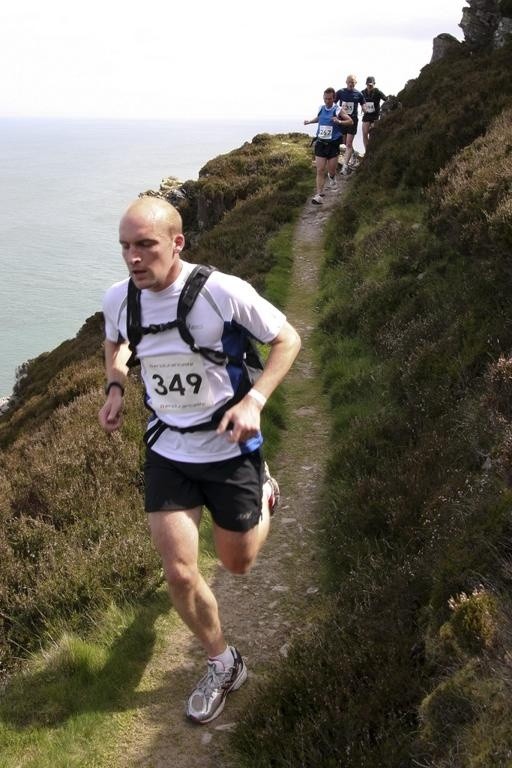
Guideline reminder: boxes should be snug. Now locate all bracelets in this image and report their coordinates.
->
[248,388,267,406]
[104,381,125,397]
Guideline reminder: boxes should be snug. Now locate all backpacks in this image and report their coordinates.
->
[126,263,265,395]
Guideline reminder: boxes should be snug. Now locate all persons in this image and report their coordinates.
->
[304,74,387,204]
[96,193,302,724]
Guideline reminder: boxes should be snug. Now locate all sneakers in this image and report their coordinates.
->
[261,460,280,518]
[328,172,337,187]
[339,150,360,176]
[311,193,326,205]
[185,645,249,726]
